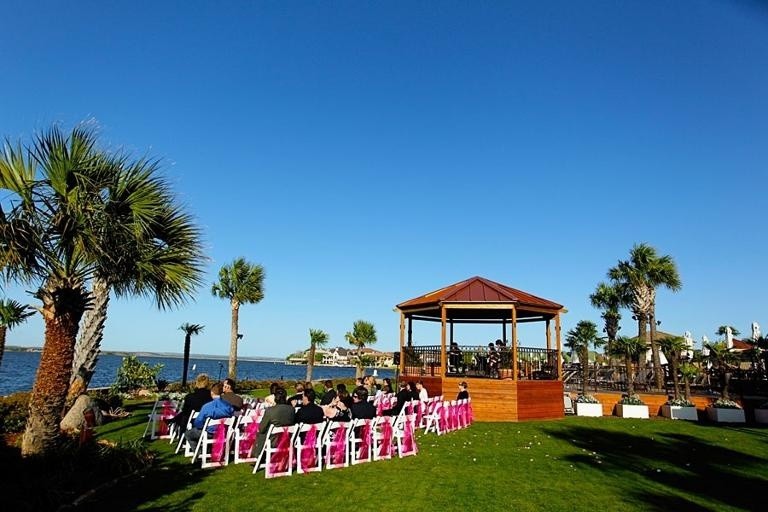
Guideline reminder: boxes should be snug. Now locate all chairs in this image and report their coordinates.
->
[313,419,355,469]
[348,418,376,465]
[226,415,263,464]
[288,420,327,474]
[252,423,299,477]
[364,393,472,434]
[364,415,397,461]
[189,416,236,469]
[447,353,458,373]
[142,393,302,460]
[387,414,419,458]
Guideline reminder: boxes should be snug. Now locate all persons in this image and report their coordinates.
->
[164,373,429,472]
[447,339,508,400]
